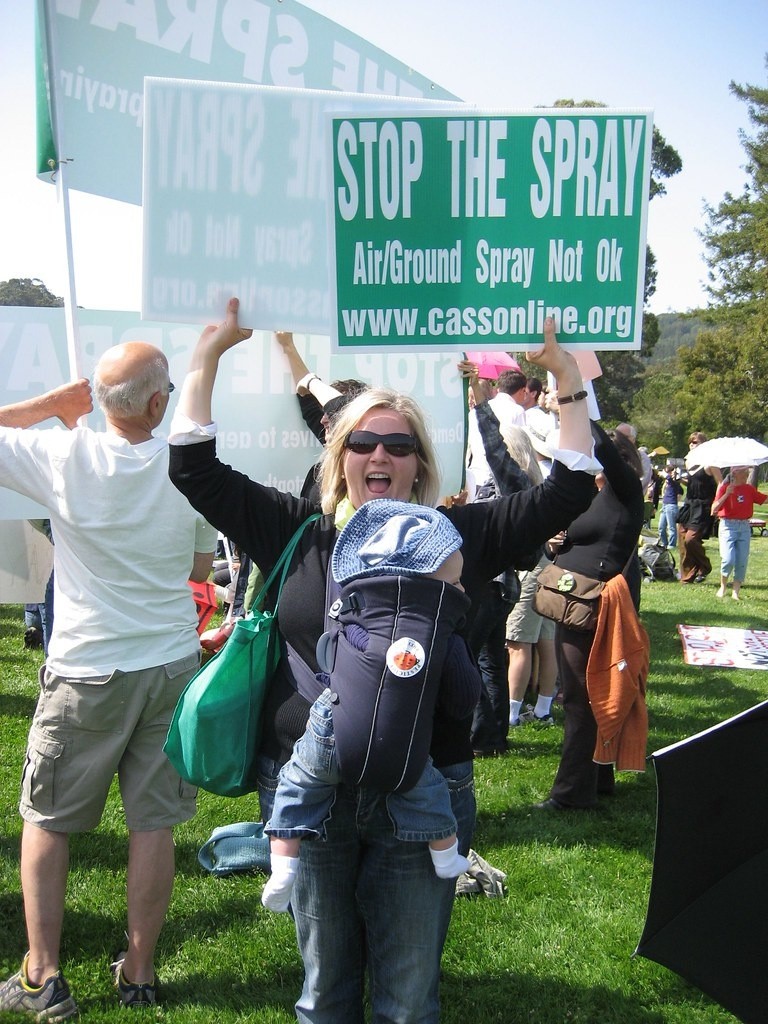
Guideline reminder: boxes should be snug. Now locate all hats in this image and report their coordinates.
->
[541,386,549,395]
[331,499,464,587]
[730,466,754,472]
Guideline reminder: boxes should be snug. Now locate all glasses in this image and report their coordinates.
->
[740,471,751,473]
[626,435,634,438]
[167,382,175,393]
[343,431,419,457]
[689,441,702,444]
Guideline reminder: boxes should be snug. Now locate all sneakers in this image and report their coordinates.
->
[521,712,555,727]
[115,951,157,1010]
[1,950,78,1023]
[509,717,521,727]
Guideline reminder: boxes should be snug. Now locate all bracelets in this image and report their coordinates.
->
[307,375,321,390]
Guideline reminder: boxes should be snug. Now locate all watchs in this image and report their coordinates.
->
[557,391,587,405]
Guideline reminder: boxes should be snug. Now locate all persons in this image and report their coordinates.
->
[0,341,218,1024]
[169,297,604,1024]
[259,497,472,911]
[25,520,55,654]
[710,466,768,602]
[456,370,644,814]
[275,328,372,504]
[605,423,724,583]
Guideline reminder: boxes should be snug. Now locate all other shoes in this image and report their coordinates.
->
[693,576,706,583]
[666,545,675,550]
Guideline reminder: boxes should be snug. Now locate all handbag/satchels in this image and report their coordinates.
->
[161,508,325,797]
[643,543,676,580]
[531,563,608,631]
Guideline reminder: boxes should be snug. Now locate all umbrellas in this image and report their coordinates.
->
[630,701,768,1024]
[684,436,768,508]
[465,351,520,368]
[465,351,521,380]
